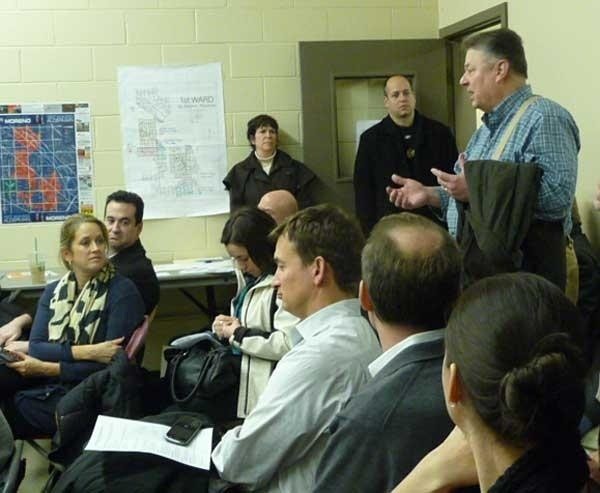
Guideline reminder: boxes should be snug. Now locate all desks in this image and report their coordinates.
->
[0,257,237,322]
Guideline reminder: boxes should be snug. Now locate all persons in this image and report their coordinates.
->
[42,187,600,493]
[384,29,581,297]
[222,113,318,212]
[352,73,459,239]
[2,187,159,492]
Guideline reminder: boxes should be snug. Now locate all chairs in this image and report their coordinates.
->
[2,303,159,493]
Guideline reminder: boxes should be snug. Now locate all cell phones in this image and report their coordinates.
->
[164,414,203,445]
[0,347,24,363]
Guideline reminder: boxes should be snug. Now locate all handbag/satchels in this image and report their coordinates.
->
[565,240,579,306]
[164,339,241,411]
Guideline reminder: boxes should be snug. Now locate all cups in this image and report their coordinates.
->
[26,249,48,285]
